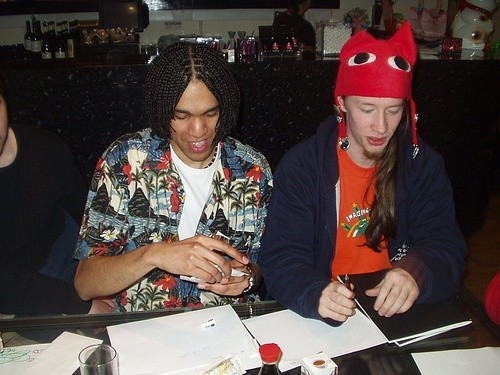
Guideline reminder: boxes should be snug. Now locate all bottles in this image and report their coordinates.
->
[26,20,80,61]
[258,344,283,375]
[261,25,300,56]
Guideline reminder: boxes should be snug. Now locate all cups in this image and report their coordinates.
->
[79,343,119,375]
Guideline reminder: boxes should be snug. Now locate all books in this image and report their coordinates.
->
[336,267,473,348]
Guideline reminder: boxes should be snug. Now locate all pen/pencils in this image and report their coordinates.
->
[345,274,350,289]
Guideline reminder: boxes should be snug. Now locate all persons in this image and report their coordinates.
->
[72,41,273,315]
[272,0,316,54]
[257,21,469,327]
[0,94,92,315]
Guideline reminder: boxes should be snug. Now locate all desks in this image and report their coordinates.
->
[0,285,500,375]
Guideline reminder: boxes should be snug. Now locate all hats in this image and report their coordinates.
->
[334,22,419,159]
